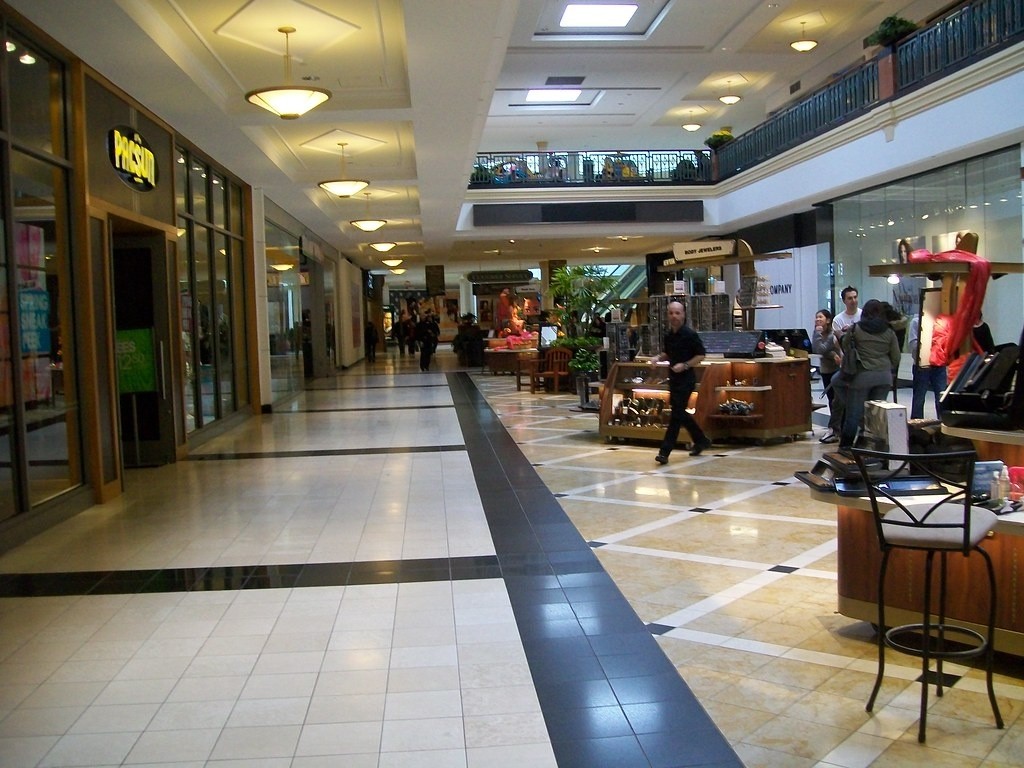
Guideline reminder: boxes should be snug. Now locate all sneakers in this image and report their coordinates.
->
[819,427,839,443]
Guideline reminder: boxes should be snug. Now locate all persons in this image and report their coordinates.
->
[812,286,994,450]
[590,313,607,339]
[364,321,379,363]
[887,239,923,318]
[391,309,441,372]
[649,301,712,464]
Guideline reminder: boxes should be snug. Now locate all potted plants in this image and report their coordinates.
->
[703,130,735,149]
[868,14,918,45]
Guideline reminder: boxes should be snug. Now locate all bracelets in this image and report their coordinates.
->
[657,354,662,361]
[684,362,688,370]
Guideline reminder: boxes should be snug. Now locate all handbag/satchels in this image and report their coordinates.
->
[840,323,858,383]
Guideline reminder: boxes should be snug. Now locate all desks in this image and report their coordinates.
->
[482,338,540,376]
[515,358,549,394]
[809,422,1024,668]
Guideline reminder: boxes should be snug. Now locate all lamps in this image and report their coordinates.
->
[381,252,403,267]
[717,80,741,105]
[790,22,818,52]
[269,242,294,271]
[244,27,333,120]
[317,143,370,198]
[350,193,387,232]
[368,228,397,252]
[681,111,701,132]
[388,267,408,275]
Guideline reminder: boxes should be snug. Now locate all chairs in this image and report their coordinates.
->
[850,447,1005,743]
[530,347,573,395]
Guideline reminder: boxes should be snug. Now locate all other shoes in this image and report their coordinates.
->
[689,437,711,456]
[655,453,669,464]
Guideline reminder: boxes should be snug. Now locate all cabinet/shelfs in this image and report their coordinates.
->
[599,362,772,444]
